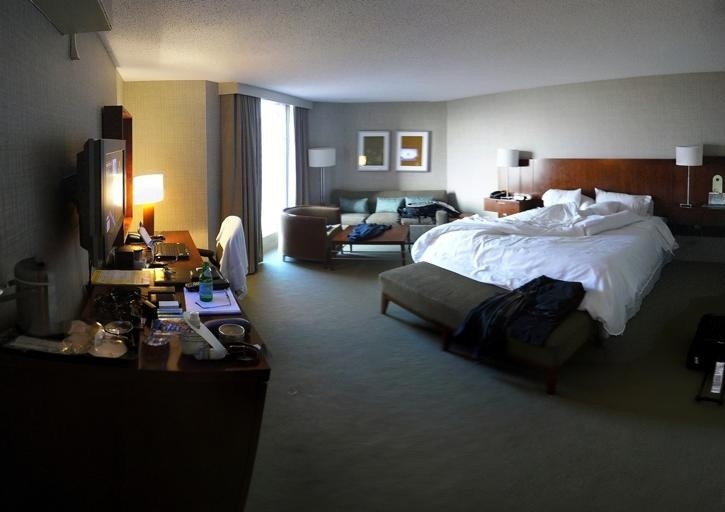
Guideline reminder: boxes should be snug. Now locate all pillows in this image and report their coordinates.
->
[405,196,434,208]
[339,196,369,213]
[595,187,652,219]
[543,188,582,211]
[375,196,404,214]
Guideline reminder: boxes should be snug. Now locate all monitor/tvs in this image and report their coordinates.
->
[75,138,129,263]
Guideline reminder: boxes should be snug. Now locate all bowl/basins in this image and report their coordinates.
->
[218,324,245,340]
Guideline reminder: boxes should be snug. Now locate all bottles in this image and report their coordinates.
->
[200,263,213,302]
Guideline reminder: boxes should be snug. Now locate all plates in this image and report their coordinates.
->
[105,320,133,335]
[86,340,129,358]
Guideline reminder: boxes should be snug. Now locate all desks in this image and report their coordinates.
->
[0,230,270,512]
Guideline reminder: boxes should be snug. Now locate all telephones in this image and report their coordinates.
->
[490,190,510,198]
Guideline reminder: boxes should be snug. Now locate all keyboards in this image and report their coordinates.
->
[152,240,192,260]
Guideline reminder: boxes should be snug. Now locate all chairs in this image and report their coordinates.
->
[195,216,242,299]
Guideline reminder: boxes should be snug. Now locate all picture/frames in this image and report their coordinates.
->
[394,130,430,173]
[356,129,390,173]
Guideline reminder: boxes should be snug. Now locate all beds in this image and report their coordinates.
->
[380,188,676,392]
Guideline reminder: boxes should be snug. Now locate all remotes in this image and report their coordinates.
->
[184,279,230,290]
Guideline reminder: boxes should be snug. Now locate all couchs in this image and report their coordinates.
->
[326,189,450,244]
[279,204,344,269]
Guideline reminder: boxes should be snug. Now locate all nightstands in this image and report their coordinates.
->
[484,195,541,219]
[674,205,724,283]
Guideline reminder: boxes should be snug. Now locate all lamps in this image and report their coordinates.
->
[133,173,164,237]
[308,146,336,205]
[675,144,704,210]
[497,148,520,195]
